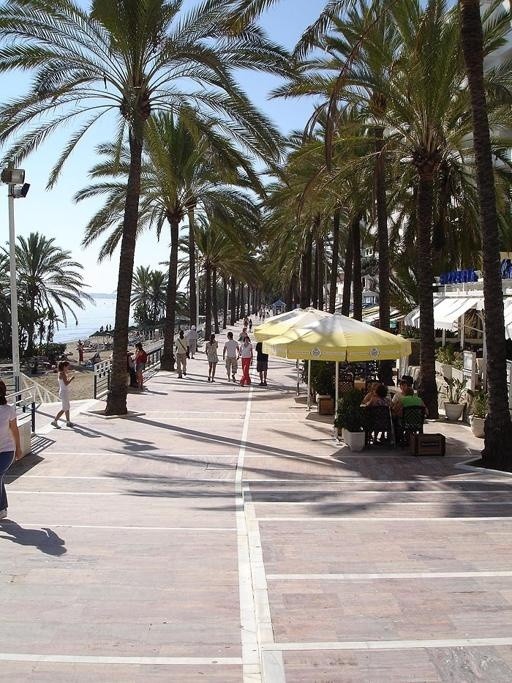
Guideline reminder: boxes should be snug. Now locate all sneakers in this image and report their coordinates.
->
[51,421,77,429]
[0,508,7,520]
[207,374,268,387]
[177,372,186,378]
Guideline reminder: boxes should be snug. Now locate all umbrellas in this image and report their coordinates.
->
[89,317,190,346]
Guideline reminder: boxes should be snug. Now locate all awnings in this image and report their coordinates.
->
[321,305,407,328]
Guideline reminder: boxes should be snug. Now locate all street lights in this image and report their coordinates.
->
[227,278,233,313]
[2,159,36,408]
[193,254,204,332]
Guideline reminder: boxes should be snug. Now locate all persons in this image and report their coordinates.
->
[244,315,252,333]
[127,342,146,392]
[364,375,429,444]
[175,325,199,379]
[0,378,23,518]
[205,334,218,383]
[237,336,269,386]
[223,332,239,381]
[239,328,248,343]
[51,360,76,429]
[254,306,270,323]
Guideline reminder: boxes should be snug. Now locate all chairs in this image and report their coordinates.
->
[330,364,432,453]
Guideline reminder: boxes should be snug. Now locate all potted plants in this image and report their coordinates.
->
[438,376,468,423]
[313,361,337,419]
[465,387,488,437]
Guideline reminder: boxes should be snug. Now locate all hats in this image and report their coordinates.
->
[397,375,415,384]
